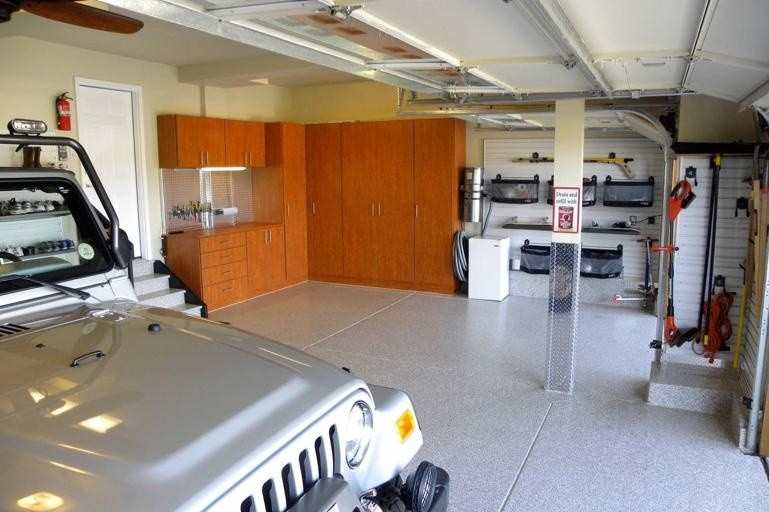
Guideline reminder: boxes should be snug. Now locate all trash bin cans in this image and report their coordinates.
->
[467,234,512,302]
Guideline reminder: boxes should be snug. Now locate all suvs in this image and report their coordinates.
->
[1,118,450,512]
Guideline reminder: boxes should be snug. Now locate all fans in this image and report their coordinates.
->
[0,0,144,35]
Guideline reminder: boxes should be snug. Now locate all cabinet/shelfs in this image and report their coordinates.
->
[157,114,225,168]
[224,118,266,168]
[165,232,247,313]
[414,117,467,294]
[0,210,81,266]
[340,120,414,290]
[306,123,344,284]
[252,122,308,285]
[502,157,641,235]
[246,226,287,300]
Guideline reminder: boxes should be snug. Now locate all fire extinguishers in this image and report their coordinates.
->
[56,91,73,131]
